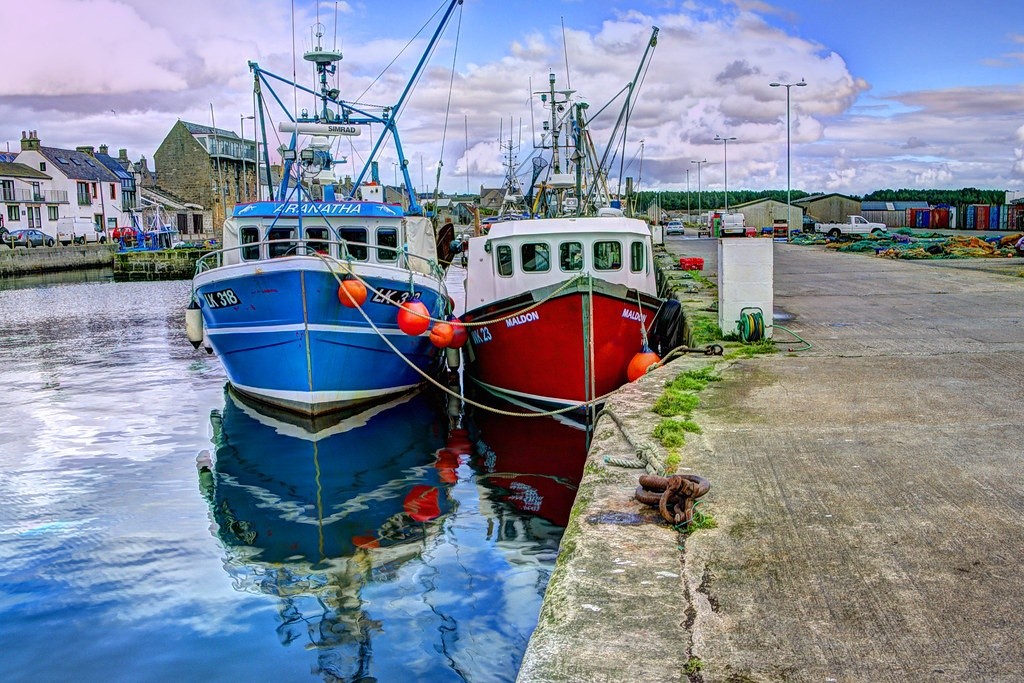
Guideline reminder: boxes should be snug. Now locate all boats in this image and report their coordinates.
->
[185,0,463,420]
[457,16,680,418]
[196,386,460,683]
[463,399,592,601]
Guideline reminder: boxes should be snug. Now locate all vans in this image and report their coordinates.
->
[57,216,106,246]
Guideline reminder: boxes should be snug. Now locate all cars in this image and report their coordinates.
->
[5,229,55,248]
[112,227,150,243]
[667,220,684,235]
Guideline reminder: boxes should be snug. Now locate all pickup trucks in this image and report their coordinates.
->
[814,215,887,238]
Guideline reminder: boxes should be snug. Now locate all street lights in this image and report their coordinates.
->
[691,158,707,224]
[239,113,255,199]
[648,191,662,225]
[769,77,808,242]
[713,133,737,213]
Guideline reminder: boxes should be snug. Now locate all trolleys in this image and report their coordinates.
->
[697,227,711,238]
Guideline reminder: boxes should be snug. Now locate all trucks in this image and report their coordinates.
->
[718,213,746,237]
